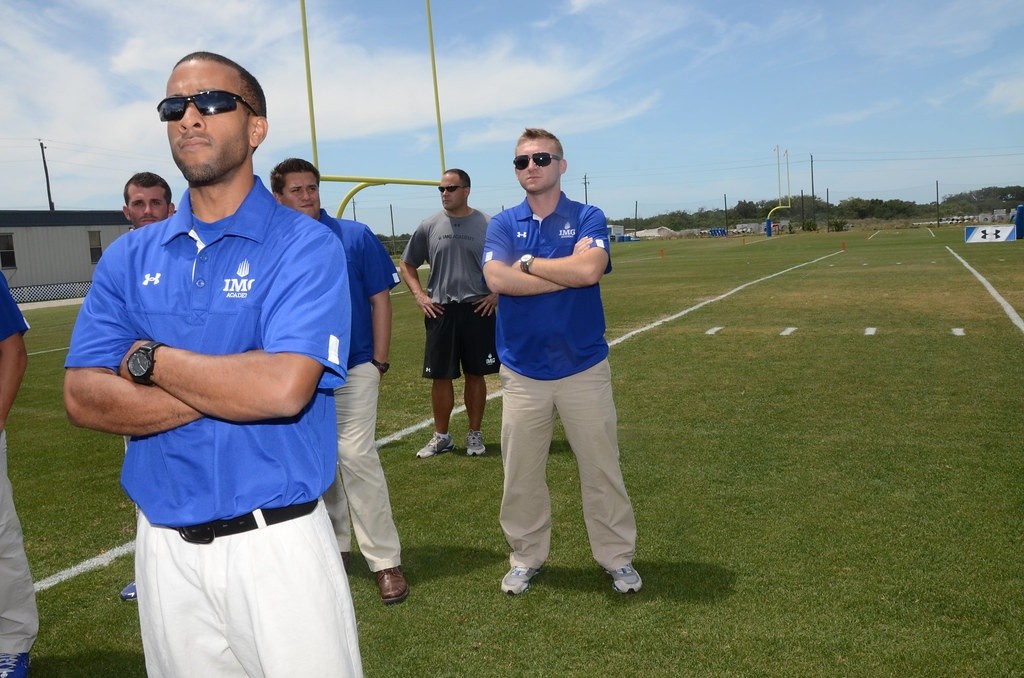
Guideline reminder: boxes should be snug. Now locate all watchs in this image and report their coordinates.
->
[519,254,534,273]
[126,341,170,385]
[371,359,389,373]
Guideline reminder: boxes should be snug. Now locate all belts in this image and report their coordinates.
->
[168,498,319,542]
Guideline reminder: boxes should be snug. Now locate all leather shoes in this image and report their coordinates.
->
[342,552,351,571]
[376,567,409,604]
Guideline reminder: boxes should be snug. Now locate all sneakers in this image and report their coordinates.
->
[416,432,455,458]
[119,581,137,601]
[500,567,539,595]
[465,429,486,456]
[0,652,31,678]
[611,563,642,594]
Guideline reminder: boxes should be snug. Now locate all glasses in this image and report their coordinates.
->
[513,153,560,170]
[438,186,462,193]
[157,90,258,122]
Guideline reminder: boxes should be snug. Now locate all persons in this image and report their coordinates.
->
[0,271,39,678]
[270,158,408,601]
[120,172,174,601]
[399,169,501,458]
[64,52,363,678]
[482,128,641,595]
